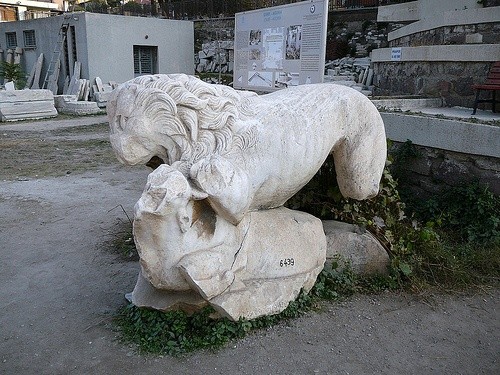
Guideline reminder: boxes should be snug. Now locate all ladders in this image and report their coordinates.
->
[42,0,76,90]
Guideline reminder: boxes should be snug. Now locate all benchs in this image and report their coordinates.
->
[471,61,500,115]
